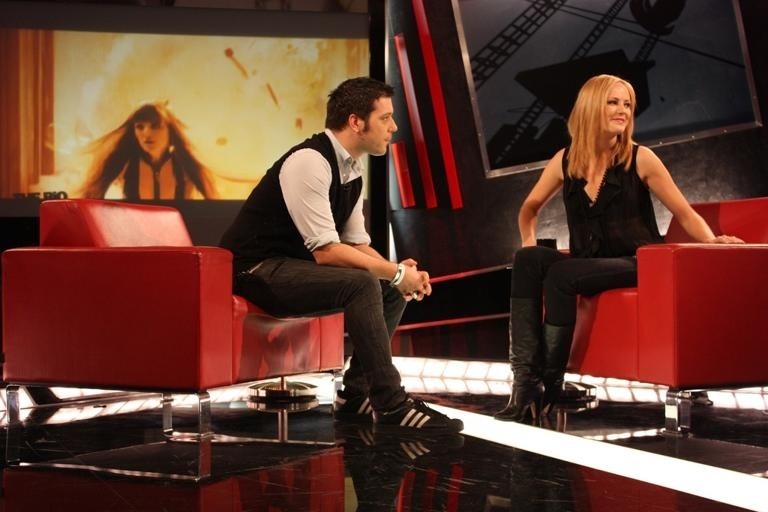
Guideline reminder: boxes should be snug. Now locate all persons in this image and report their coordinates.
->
[496,411,590,510]
[77,99,223,203]
[213,75,466,443]
[329,445,467,509]
[493,73,747,419]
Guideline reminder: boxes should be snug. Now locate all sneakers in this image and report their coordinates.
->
[376,393,465,434]
[334,387,374,422]
[338,425,466,474]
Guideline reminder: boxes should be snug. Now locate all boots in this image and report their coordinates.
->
[494,297,544,421]
[536,324,576,416]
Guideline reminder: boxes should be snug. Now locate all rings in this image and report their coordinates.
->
[412,292,417,299]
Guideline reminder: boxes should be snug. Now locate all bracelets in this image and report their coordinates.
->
[387,262,405,291]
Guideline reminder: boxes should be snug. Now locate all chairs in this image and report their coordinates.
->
[569,195,768,437]
[0,197,349,485]
